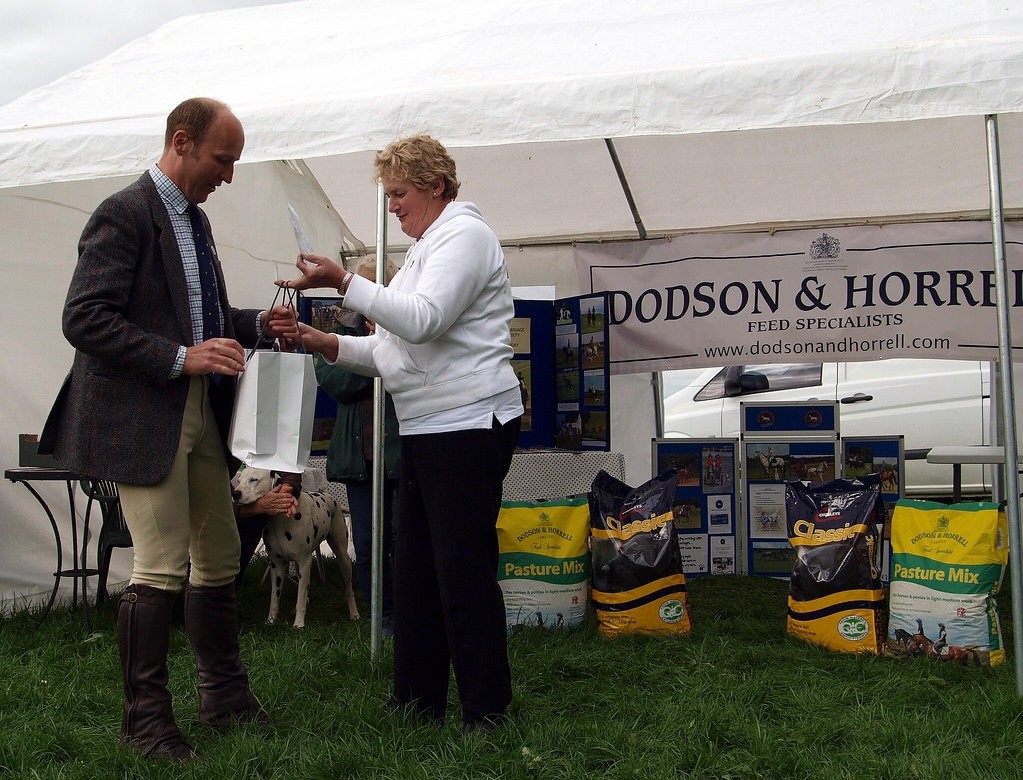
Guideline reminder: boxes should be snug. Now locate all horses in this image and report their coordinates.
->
[326,308,341,329]
[668,409,899,577]
[894,626,982,670]
[517,306,605,445]
[512,624,550,641]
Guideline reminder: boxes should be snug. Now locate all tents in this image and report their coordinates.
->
[0,4,1023,705]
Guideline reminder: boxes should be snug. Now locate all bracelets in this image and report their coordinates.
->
[337,270,354,296]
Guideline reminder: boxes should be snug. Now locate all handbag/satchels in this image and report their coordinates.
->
[226,279,318,475]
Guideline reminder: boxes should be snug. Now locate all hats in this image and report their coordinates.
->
[916,618,922,623]
[715,454,720,458]
[937,623,946,628]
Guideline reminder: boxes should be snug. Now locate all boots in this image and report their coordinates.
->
[115,581,267,763]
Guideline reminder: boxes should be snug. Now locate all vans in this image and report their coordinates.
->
[663,359,992,497]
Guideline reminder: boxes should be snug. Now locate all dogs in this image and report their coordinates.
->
[229,465,362,631]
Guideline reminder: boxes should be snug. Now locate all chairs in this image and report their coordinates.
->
[79,479,134,616]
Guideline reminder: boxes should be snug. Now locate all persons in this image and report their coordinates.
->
[706,452,722,478]
[590,336,595,356]
[765,445,775,470]
[777,546,788,560]
[587,305,596,315]
[35,98,300,768]
[314,254,406,622]
[592,383,595,394]
[567,338,571,350]
[793,459,802,478]
[881,458,889,472]
[516,371,524,382]
[272,132,527,718]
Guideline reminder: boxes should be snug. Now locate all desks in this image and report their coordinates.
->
[4,466,99,632]
[926,446,1023,506]
